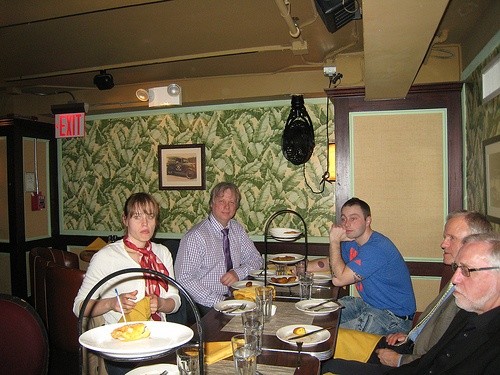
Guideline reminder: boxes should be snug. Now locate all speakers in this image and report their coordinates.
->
[314,0,356,34]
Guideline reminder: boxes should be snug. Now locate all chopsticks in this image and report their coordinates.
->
[307,298,337,309]
[290,326,334,339]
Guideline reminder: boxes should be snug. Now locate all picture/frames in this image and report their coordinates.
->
[158,143,207,191]
[481,134,500,224]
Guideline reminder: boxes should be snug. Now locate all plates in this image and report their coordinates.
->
[124,363,181,375]
[232,280,264,290]
[312,273,334,284]
[78,321,194,358]
[268,275,302,287]
[269,253,306,264]
[295,299,340,316]
[214,300,257,315]
[270,227,302,241]
[251,271,276,280]
[275,324,331,348]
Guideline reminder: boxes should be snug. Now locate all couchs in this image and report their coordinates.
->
[29,246,87,353]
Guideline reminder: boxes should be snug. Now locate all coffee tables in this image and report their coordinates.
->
[78,249,98,263]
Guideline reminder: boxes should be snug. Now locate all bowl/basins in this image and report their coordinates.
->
[264,304,276,316]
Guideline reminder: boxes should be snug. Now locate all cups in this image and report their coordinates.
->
[254,285,273,324]
[175,342,206,375]
[299,271,313,300]
[241,310,264,353]
[231,334,259,375]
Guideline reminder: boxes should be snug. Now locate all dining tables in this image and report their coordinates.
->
[136,276,343,375]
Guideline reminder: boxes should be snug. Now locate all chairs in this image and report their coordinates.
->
[0,292,50,375]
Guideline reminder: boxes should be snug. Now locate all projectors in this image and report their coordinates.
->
[95,76,114,90]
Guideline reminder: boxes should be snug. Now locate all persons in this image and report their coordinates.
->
[300,198,417,333]
[73,192,181,375]
[174,181,263,319]
[321,209,500,375]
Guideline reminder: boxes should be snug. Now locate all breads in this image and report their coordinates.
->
[293,327,306,335]
[272,256,296,261]
[246,282,252,287]
[271,276,296,284]
[111,323,149,341]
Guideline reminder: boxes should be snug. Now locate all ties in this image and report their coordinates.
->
[221,228,236,298]
[392,281,454,346]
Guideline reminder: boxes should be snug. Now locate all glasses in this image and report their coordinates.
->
[218,200,235,207]
[451,262,500,277]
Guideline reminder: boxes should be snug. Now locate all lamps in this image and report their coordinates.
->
[93,70,115,90]
[314,0,362,34]
[135,84,183,109]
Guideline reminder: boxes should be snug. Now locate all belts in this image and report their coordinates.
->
[397,314,416,322]
